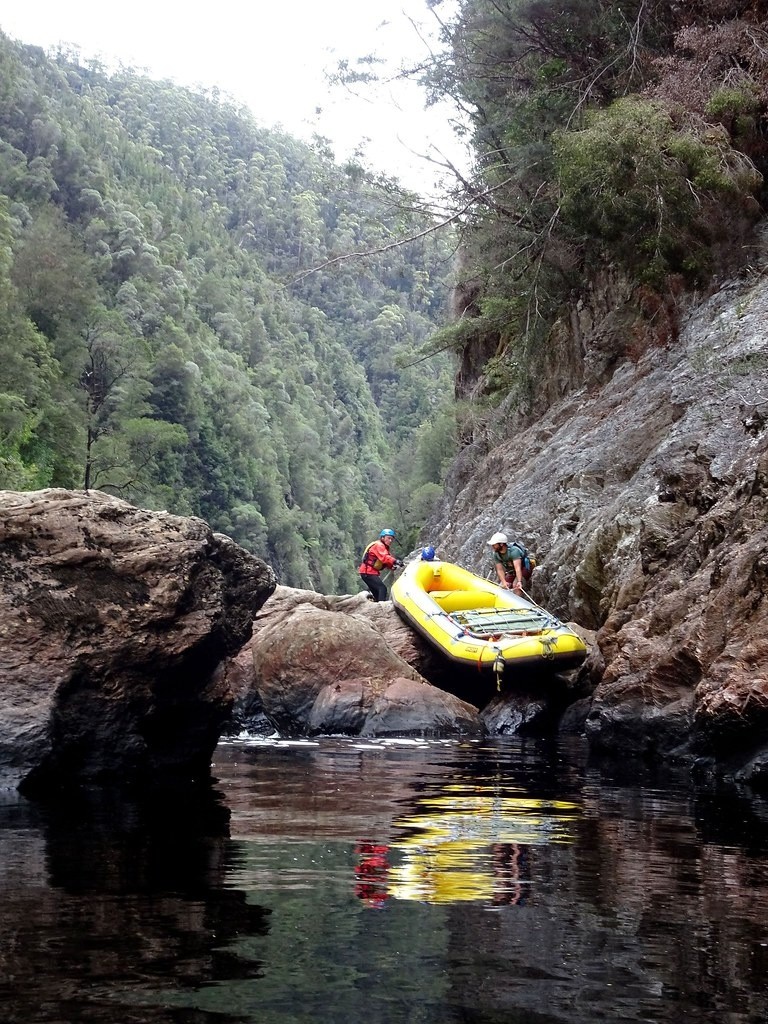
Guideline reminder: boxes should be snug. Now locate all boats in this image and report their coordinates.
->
[392,549,585,679]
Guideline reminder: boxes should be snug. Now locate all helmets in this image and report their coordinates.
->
[487,532,508,545]
[380,529,396,538]
[422,546,434,560]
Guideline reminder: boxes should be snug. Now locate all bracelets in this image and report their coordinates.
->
[518,581,522,582]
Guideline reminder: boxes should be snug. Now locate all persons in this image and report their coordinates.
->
[359,529,403,602]
[416,546,440,561]
[487,533,532,597]
